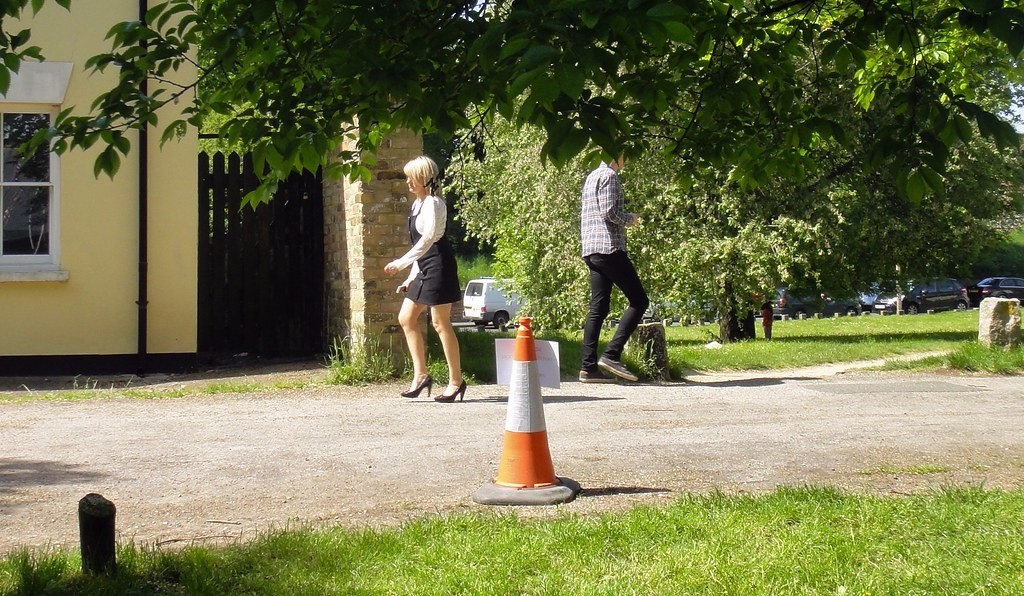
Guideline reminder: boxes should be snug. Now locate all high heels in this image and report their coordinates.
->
[401,374,433,398]
[434,379,467,402]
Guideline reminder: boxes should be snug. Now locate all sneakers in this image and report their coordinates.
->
[579,368,617,382]
[597,354,638,381]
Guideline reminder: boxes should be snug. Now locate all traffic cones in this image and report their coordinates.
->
[471,317,582,507]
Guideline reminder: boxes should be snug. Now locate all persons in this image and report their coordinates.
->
[579,152,650,383]
[384,156,466,403]
[763,302,772,339]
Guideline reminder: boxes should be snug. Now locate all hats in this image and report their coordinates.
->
[761,299,773,309]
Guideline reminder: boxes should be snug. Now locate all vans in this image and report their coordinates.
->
[462,277,528,329]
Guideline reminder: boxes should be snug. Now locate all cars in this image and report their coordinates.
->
[872,278,970,315]
[967,277,1024,308]
[769,284,861,320]
